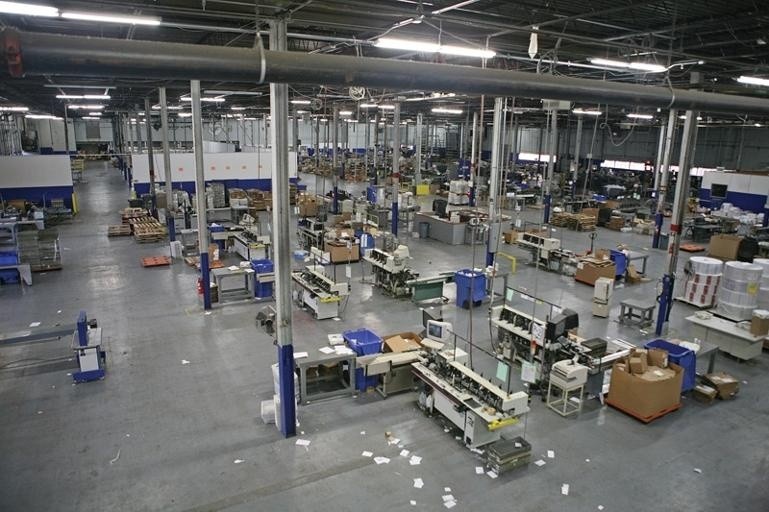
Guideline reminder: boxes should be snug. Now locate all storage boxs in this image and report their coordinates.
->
[250,260,272,298]
[751,306,769,337]
[344,329,424,393]
[606,336,740,425]
[709,233,744,260]
[577,249,645,286]
[578,202,626,231]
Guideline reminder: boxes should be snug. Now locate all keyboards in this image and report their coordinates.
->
[420,337,444,350]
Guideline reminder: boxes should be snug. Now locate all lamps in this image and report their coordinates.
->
[591,58,665,73]
[736,75,769,86]
[60,11,161,28]
[374,39,497,59]
[1,2,59,17]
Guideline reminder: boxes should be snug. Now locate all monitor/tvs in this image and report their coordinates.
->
[242,212,256,223]
[392,244,411,262]
[710,182,728,199]
[561,308,579,336]
[546,314,566,339]
[422,308,443,327]
[426,320,453,342]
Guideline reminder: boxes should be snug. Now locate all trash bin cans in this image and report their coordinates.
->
[419,222,428,238]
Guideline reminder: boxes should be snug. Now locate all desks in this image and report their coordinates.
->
[210,266,254,303]
[294,337,357,406]
